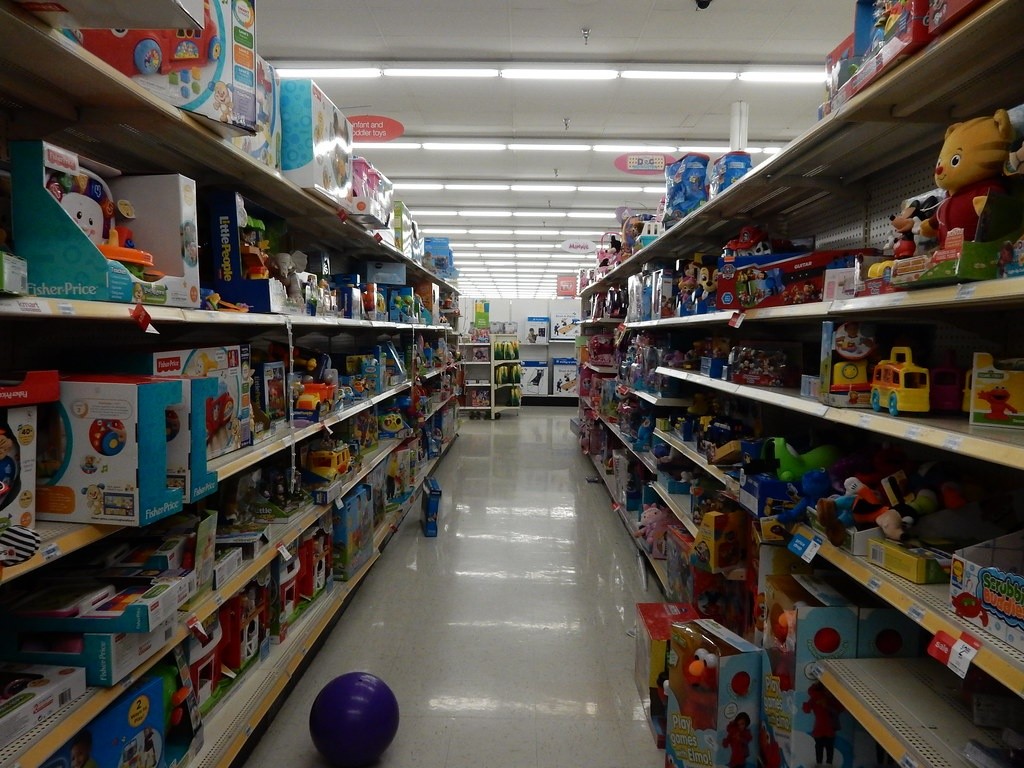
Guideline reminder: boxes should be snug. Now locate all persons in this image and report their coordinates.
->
[526,328,536,343]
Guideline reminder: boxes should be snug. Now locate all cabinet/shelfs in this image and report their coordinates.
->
[517,339,580,406]
[567,0,1024,768]
[0,0,470,768]
[460,333,523,420]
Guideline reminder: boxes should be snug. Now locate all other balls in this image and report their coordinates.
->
[306,670,401,768]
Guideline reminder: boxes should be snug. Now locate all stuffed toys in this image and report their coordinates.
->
[921,109,1017,249]
[693,255,718,306]
[678,275,697,302]
[774,600,806,689]
[605,235,621,254]
[679,647,719,730]
[636,400,661,443]
[773,462,979,547]
[634,503,662,553]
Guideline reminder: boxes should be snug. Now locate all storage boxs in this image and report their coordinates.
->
[525,315,549,344]
[551,358,578,398]
[0,0,463,768]
[579,0,1024,768]
[519,359,548,397]
[550,312,581,340]
